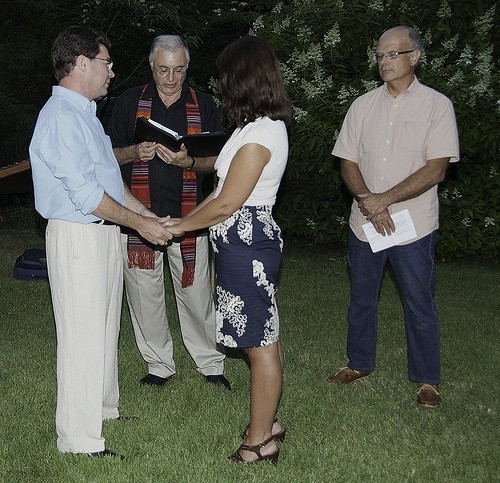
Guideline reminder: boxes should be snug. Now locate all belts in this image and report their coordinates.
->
[92,219,119,226]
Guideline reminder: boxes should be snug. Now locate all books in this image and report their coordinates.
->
[133,116,228,158]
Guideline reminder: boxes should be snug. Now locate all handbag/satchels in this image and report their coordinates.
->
[13,249,48,280]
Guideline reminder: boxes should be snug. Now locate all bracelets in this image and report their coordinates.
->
[186,157,195,171]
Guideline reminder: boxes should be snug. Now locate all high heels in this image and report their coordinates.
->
[240,418,287,444]
[225,435,280,467]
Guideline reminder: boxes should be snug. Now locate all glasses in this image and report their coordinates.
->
[373,49,414,61]
[89,56,114,70]
[152,63,187,76]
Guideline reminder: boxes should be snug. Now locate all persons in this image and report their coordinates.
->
[161,34,294,467]
[327,25,460,409]
[29,25,173,461]
[104,32,232,390]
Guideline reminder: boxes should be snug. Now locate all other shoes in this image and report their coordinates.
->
[139,374,174,388]
[325,367,371,386]
[71,449,125,461]
[203,375,232,391]
[112,417,139,421]
[414,383,442,409]
